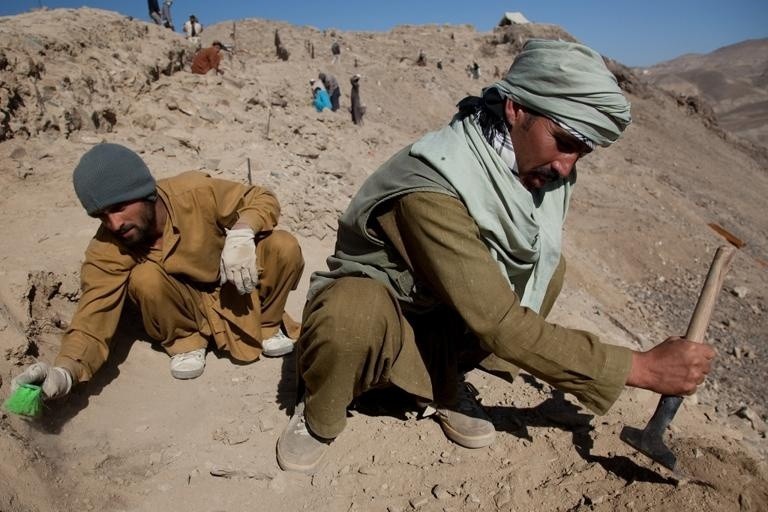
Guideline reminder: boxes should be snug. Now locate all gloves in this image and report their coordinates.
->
[11,362,73,421]
[219,227,258,295]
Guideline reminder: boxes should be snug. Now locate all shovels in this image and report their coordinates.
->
[616,245,736,483]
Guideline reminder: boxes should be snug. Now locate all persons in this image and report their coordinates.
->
[148,0,161,25]
[417,49,426,67]
[472,60,479,79]
[11,148,305,407]
[493,66,500,79]
[183,16,203,39]
[191,40,225,75]
[437,60,442,69]
[309,73,340,112]
[465,65,474,75]
[163,1,174,32]
[350,74,363,125]
[276,37,716,472]
[331,37,340,64]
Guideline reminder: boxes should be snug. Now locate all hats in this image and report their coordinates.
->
[74,144,158,218]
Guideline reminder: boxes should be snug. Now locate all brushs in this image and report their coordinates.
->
[2,383,43,418]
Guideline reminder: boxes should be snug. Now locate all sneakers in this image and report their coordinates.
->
[262,328,294,356]
[423,382,496,448]
[170,348,206,378]
[277,394,334,472]
